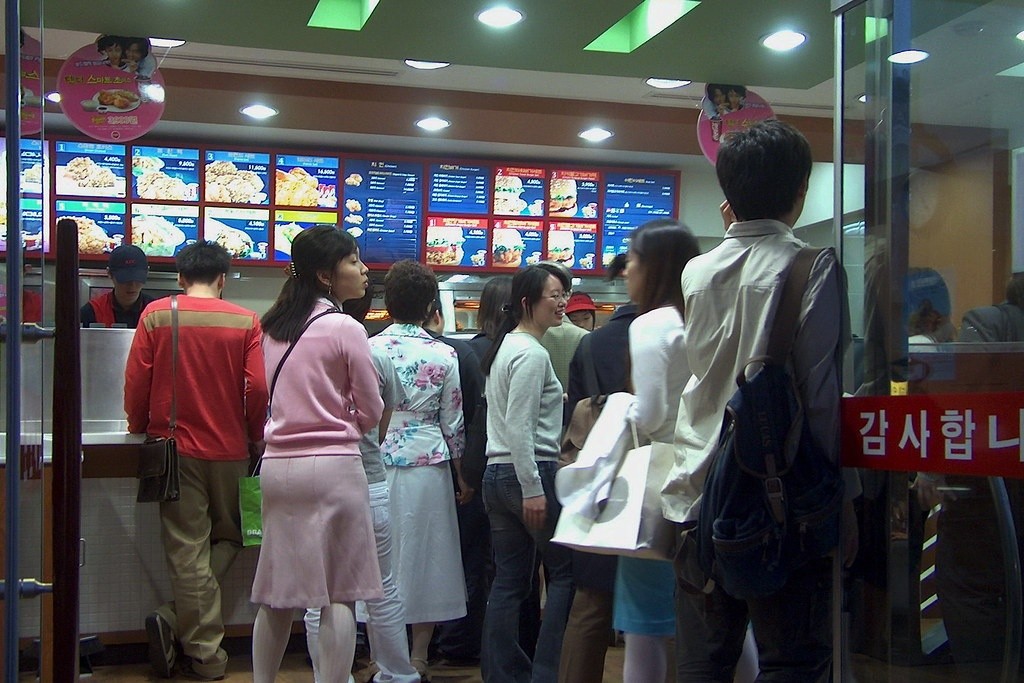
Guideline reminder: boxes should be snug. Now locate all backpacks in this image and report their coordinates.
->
[704,247,840,621]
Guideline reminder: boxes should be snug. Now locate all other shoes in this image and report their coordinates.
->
[409,658,432,683]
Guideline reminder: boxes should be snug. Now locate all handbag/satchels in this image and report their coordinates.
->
[135,436,180,503]
[553,424,674,561]
[239,457,266,545]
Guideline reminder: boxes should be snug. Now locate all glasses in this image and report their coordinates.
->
[540,293,568,301]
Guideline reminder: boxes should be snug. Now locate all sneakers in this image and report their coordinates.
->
[175,657,225,681]
[145,612,178,678]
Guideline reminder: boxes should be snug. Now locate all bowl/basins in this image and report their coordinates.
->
[96,106,109,114]
[21,100,26,108]
[80,100,99,112]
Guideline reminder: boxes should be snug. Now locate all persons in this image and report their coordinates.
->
[103,35,157,80]
[304,219,762,683]
[80,244,156,329]
[661,117,863,683]
[707,84,747,114]
[844,243,1024,683]
[124,240,269,681]
[250,224,384,683]
[0,256,41,322]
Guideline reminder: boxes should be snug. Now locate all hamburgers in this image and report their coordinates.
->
[547,230,575,268]
[425,226,466,266]
[494,175,527,215]
[492,228,527,267]
[549,178,578,219]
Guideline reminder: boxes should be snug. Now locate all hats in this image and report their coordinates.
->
[564,295,597,314]
[109,245,150,283]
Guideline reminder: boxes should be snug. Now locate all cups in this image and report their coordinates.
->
[534,199,544,213]
[134,76,151,103]
[258,243,268,259]
[589,202,598,211]
[532,251,542,261]
[478,250,487,266]
[585,253,595,268]
[709,118,723,142]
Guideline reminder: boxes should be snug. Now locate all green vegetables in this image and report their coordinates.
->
[137,241,176,256]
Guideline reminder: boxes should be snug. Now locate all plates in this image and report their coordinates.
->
[92,89,141,112]
[23,88,34,99]
[719,133,725,144]
